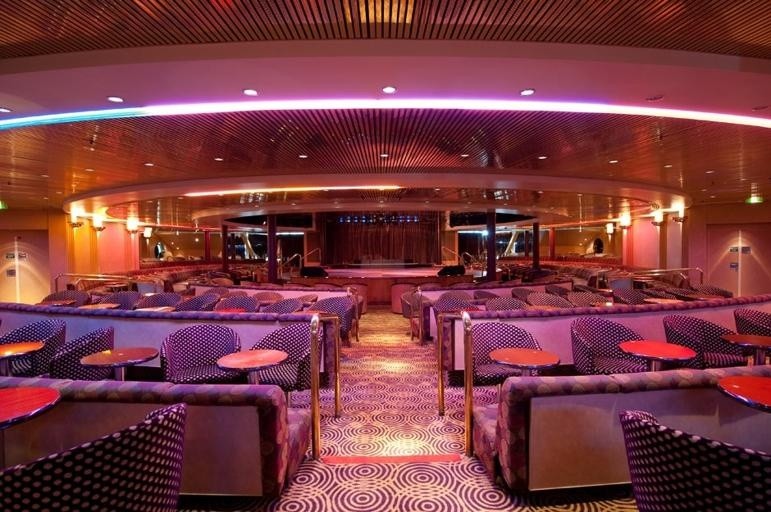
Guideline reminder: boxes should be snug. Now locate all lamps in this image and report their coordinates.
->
[69,222,83,228]
[126,229,139,234]
[673,216,686,222]
[92,226,106,230]
[651,222,665,227]
[618,226,631,229]
